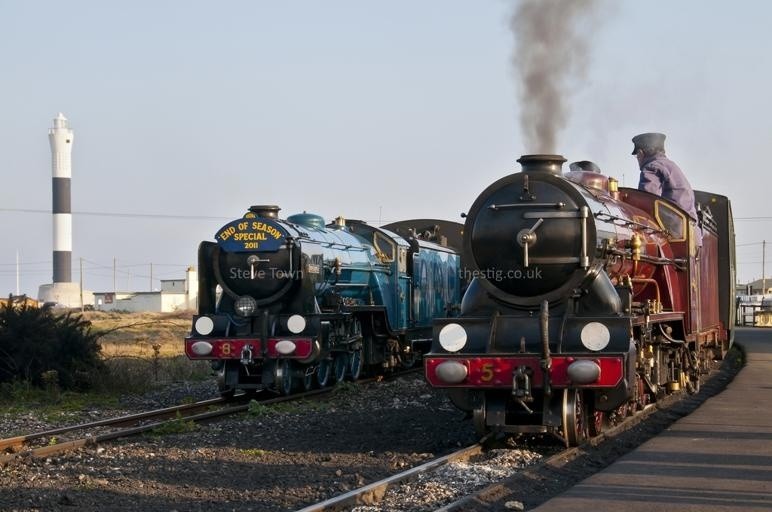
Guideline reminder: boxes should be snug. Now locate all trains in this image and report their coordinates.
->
[183,204,469,399]
[423,153,739,449]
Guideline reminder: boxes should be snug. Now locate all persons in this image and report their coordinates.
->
[632,133,703,248]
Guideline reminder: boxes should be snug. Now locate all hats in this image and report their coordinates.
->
[632,133,666,155]
